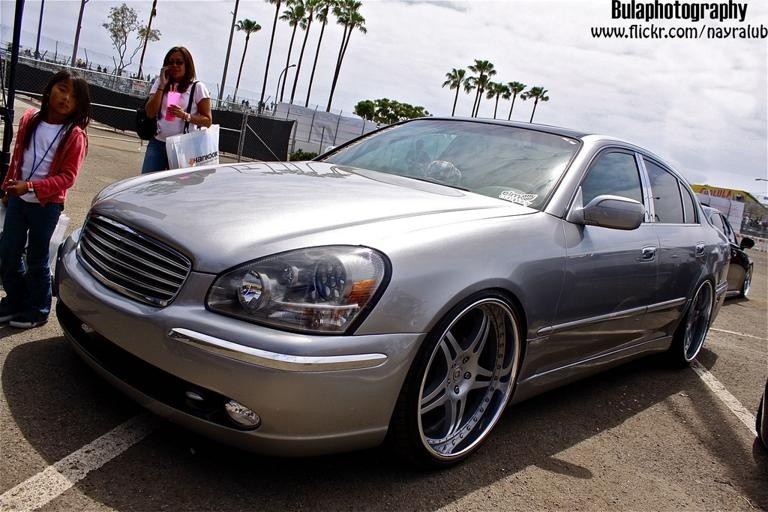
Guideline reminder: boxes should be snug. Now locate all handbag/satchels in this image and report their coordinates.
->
[134,96,159,141]
[165,80,221,170]
[0,198,70,278]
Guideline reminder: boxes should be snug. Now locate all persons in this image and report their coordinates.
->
[242,100,265,114]
[76,58,107,73]
[130,72,150,81]
[0,69,92,328]
[406,139,432,171]
[141,47,212,174]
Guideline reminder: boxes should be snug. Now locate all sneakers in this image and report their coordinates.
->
[0,298,50,330]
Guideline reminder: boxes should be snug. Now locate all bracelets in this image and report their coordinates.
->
[157,88,165,92]
[27,181,34,193]
[183,113,191,122]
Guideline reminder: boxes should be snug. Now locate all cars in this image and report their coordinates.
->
[737,216,767,238]
[697,206,756,302]
[53,116,732,470]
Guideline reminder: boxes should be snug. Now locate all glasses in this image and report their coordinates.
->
[167,58,185,66]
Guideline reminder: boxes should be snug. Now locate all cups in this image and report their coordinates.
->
[164,90,181,121]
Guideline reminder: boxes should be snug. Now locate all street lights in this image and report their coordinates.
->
[273,63,298,108]
[755,177,768,183]
